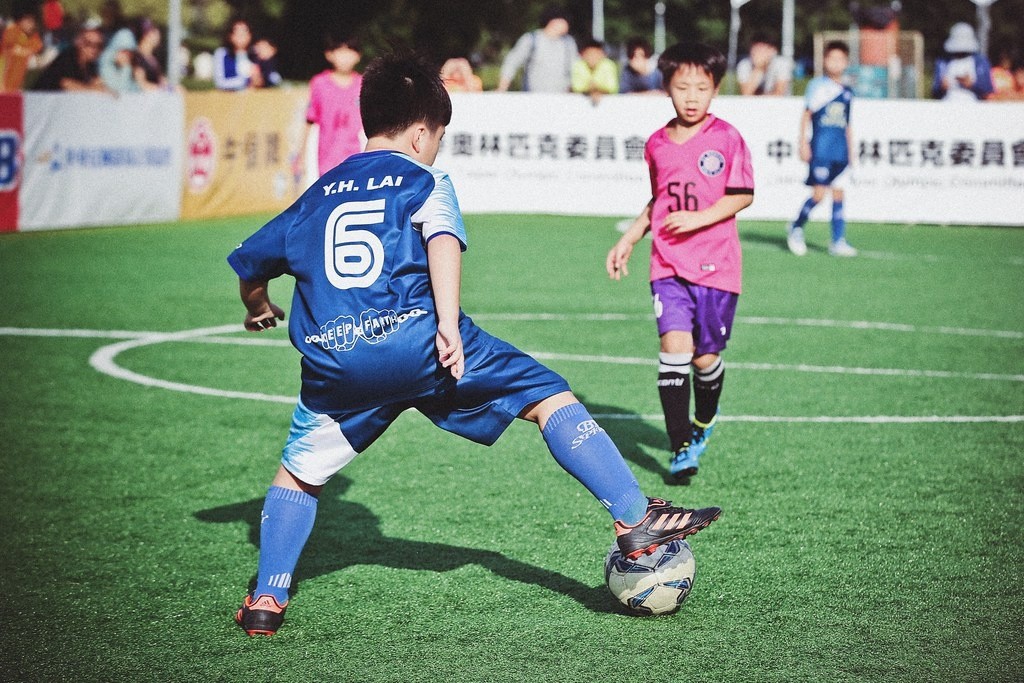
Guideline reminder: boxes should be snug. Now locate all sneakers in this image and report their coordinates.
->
[612,497,721,562]
[689,405,720,457]
[671,445,699,477]
[235,594,289,639]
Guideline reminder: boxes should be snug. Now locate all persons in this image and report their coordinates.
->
[601,44,754,485]
[0,9,1023,106]
[784,40,866,258]
[225,50,724,635]
[290,28,371,192]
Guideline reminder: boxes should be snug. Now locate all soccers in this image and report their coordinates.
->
[601,532,698,618]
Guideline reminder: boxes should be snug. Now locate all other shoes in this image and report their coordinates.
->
[788,226,807,256]
[828,238,856,258]
